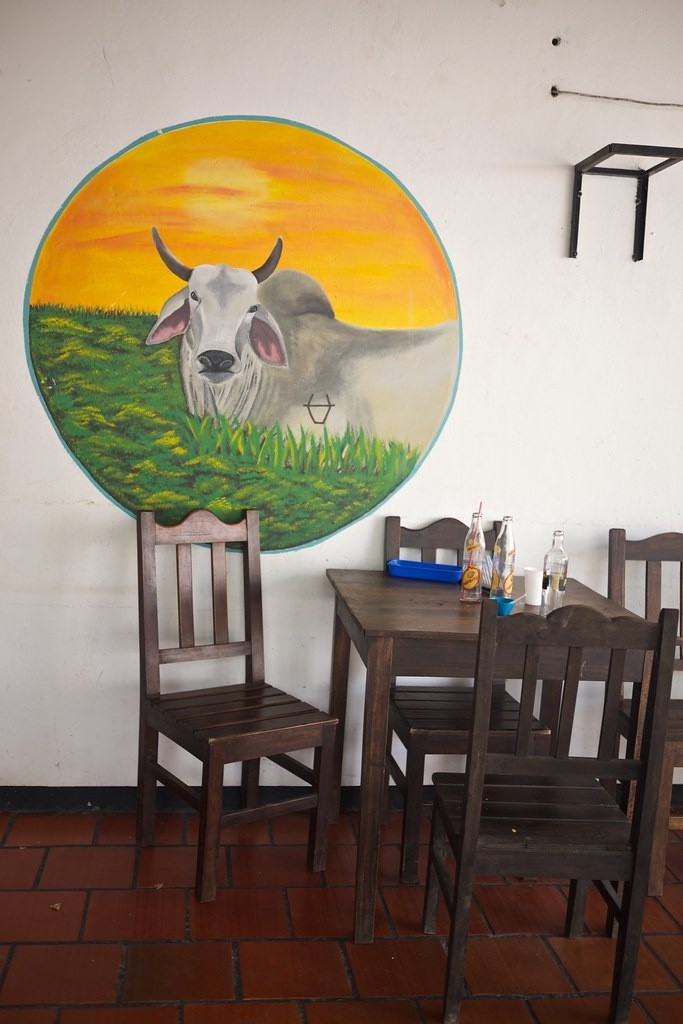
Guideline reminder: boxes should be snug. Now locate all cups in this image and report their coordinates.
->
[524,567,543,606]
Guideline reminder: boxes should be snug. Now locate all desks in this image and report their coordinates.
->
[308,569,659,945]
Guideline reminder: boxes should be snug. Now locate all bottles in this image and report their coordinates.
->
[490,516,516,599]
[539,530,568,618]
[459,513,486,603]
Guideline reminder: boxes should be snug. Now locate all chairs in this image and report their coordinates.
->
[599,527,683,895]
[424,604,677,1024]
[134,509,342,905]
[382,514,553,893]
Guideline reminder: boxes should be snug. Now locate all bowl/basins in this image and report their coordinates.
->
[489,597,515,615]
[388,559,462,584]
